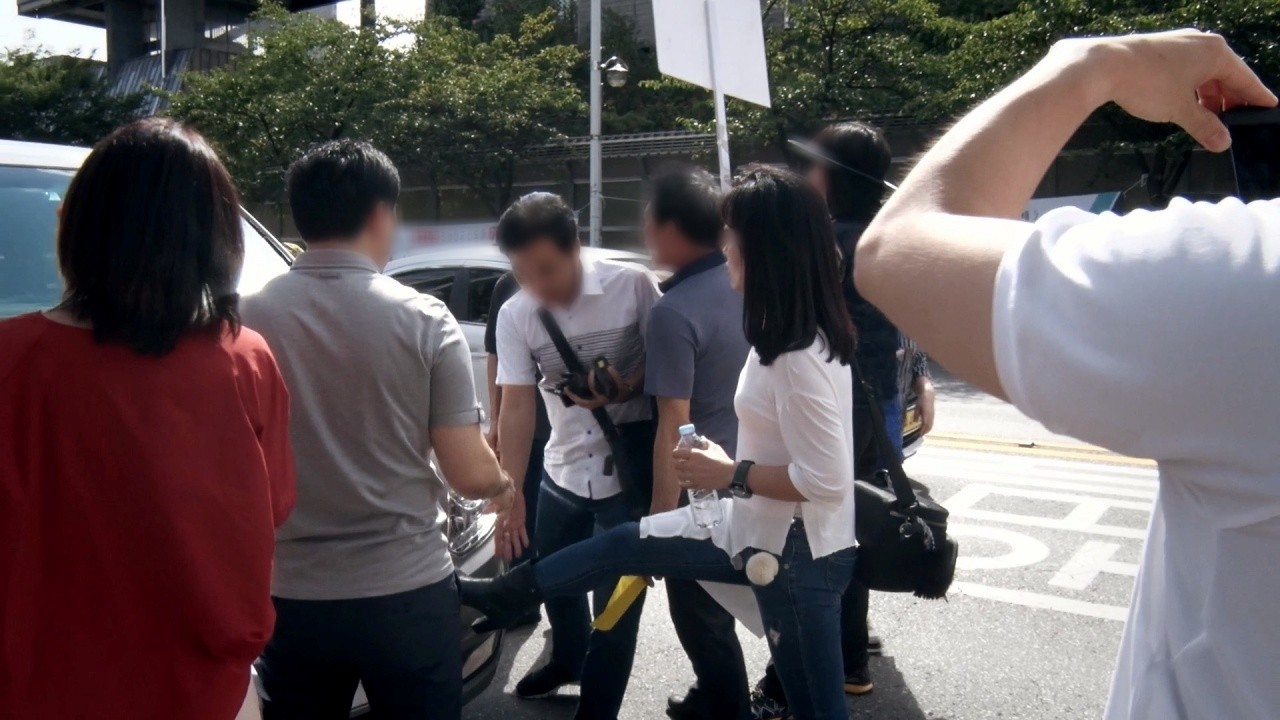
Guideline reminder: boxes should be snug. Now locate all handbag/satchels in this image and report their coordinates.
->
[855,468,957,592]
[605,420,690,517]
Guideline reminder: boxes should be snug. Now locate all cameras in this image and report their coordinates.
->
[559,357,614,407]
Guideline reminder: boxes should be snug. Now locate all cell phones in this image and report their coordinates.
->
[1223,108,1280,203]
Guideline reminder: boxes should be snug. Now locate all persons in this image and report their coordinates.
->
[456,123,930,720]
[236,138,517,720]
[0,117,299,720]
[852,29,1280,720]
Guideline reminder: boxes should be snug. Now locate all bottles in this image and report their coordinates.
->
[676,423,724,528]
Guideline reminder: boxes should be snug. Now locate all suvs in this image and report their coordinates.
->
[0,141,515,715]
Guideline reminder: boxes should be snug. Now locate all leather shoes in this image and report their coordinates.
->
[668,688,702,720]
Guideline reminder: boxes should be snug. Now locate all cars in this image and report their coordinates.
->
[365,248,676,436]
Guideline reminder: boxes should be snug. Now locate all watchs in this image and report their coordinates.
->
[728,460,756,499]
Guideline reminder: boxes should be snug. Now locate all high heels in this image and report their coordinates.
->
[460,559,543,635]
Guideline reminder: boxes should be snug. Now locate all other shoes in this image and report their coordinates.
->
[868,635,883,654]
[748,682,792,720]
[518,651,582,697]
[845,670,873,694]
